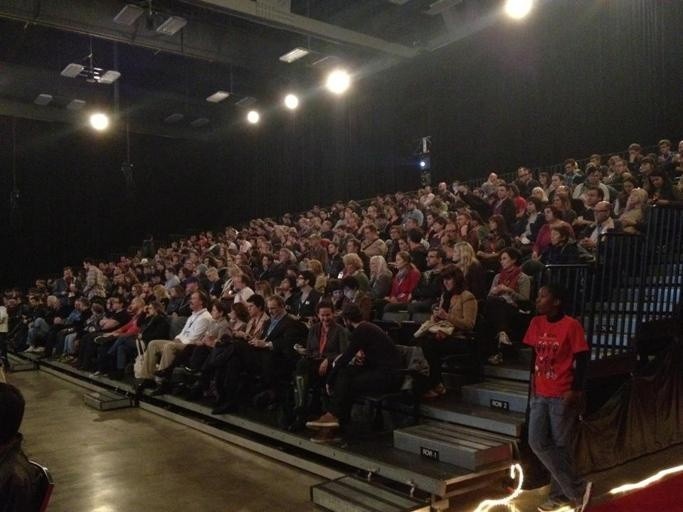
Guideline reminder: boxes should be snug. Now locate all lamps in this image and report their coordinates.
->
[157,16,186,36]
[115,4,142,25]
[68,99,87,110]
[61,63,85,79]
[326,69,349,92]
[286,94,301,110]
[34,93,55,107]
[280,46,308,65]
[98,69,122,85]
[207,90,230,104]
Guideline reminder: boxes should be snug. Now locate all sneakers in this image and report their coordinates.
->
[310,433,343,443]
[306,415,340,430]
[89,372,107,379]
[24,345,46,353]
[574,480,592,512]
[488,353,505,364]
[422,383,447,400]
[537,503,568,512]
[498,331,512,349]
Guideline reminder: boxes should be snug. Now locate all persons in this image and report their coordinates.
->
[0,381,54,512]
[523,281,593,512]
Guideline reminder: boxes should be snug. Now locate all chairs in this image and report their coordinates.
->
[155,210,623,451]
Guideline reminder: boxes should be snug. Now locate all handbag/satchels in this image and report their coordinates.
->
[414,319,455,338]
[134,353,146,378]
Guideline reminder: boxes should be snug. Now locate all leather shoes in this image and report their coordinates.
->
[211,402,238,414]
[137,364,203,397]
